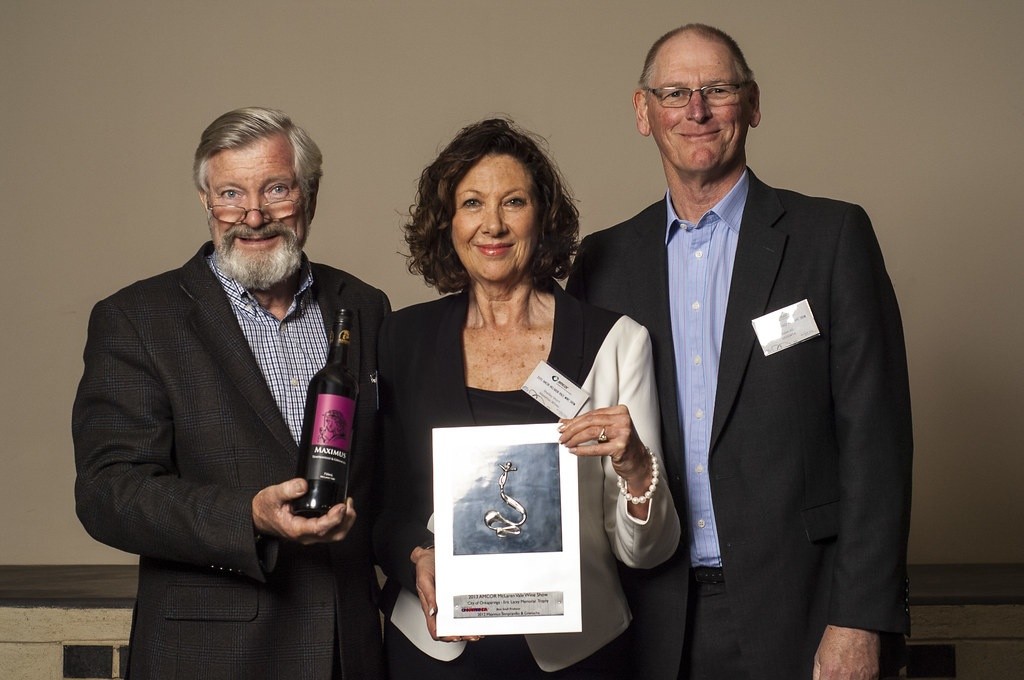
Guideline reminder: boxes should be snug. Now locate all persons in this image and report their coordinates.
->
[366,114,683,680]
[563,24,917,679]
[71,105,397,680]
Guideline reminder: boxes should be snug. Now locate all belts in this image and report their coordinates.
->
[691,567,724,583]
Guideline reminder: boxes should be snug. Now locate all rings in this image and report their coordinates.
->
[597,427,609,444]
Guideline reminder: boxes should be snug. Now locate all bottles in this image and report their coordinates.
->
[289,305,360,518]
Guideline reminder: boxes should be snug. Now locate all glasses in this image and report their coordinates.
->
[204,193,307,224]
[646,83,742,109]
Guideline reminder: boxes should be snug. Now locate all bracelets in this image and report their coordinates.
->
[615,446,660,505]
